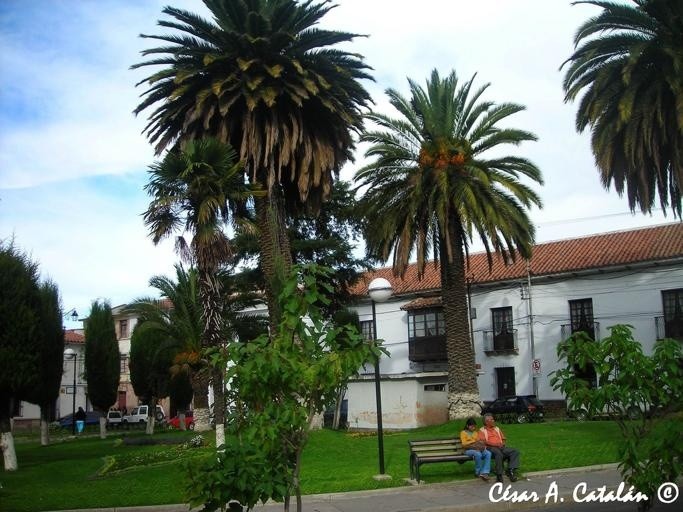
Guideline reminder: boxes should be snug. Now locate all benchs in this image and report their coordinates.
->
[407,437,510,482]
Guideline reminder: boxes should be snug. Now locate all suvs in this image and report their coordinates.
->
[123,405,165,424]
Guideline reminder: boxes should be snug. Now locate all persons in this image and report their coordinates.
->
[110,405,126,416]
[459,416,492,482]
[479,412,520,484]
[74,406,86,435]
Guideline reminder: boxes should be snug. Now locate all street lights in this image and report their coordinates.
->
[62,308,78,321]
[368,277,392,474]
[466,274,477,363]
[63,348,77,435]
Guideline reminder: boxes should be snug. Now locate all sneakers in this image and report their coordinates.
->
[474,472,518,482]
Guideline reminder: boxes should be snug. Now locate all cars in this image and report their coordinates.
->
[166,410,194,430]
[481,395,544,424]
[55,411,122,432]
[566,384,663,421]
